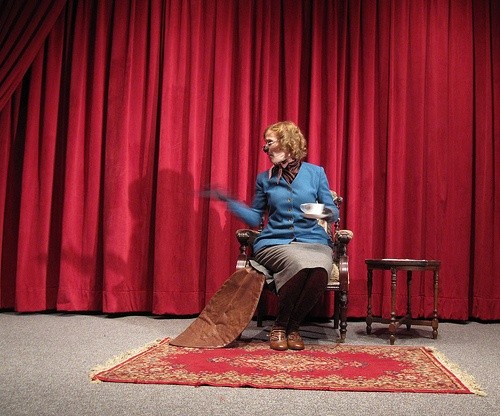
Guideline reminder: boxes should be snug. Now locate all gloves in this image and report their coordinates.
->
[205,186,230,203]
[321,208,334,223]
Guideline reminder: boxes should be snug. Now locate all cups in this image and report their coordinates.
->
[300,203,325,214]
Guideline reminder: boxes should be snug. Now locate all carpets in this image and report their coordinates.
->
[89,336,487,397]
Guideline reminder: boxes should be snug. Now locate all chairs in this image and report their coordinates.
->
[236,190,353,343]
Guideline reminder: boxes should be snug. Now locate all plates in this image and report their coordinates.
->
[302,213,329,218]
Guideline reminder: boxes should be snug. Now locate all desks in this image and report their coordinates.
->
[365,259,441,345]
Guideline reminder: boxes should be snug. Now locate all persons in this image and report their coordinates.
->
[193,121,339,351]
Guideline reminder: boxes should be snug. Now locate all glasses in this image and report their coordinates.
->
[263,137,282,146]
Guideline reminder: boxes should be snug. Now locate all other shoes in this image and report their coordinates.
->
[269,327,288,350]
[287,329,304,349]
